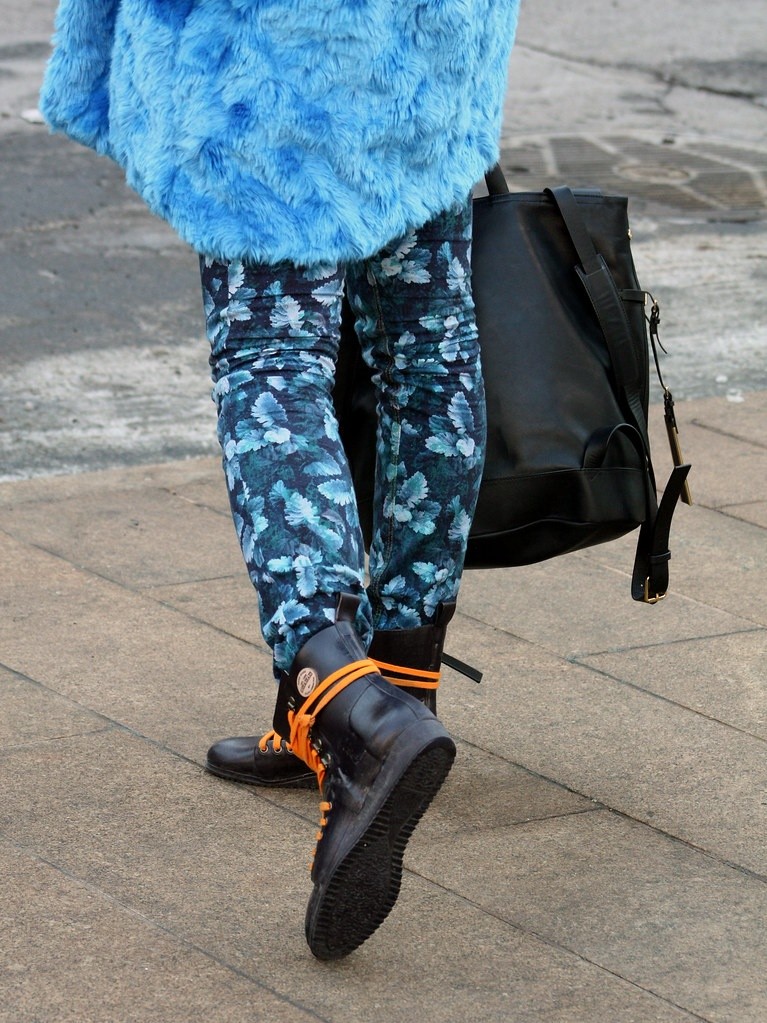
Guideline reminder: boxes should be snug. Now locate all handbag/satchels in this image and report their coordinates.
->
[334,160,691,607]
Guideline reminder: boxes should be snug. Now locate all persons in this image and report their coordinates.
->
[40,0,522,962]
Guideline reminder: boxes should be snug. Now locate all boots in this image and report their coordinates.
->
[268,588,458,964]
[206,599,454,789]
